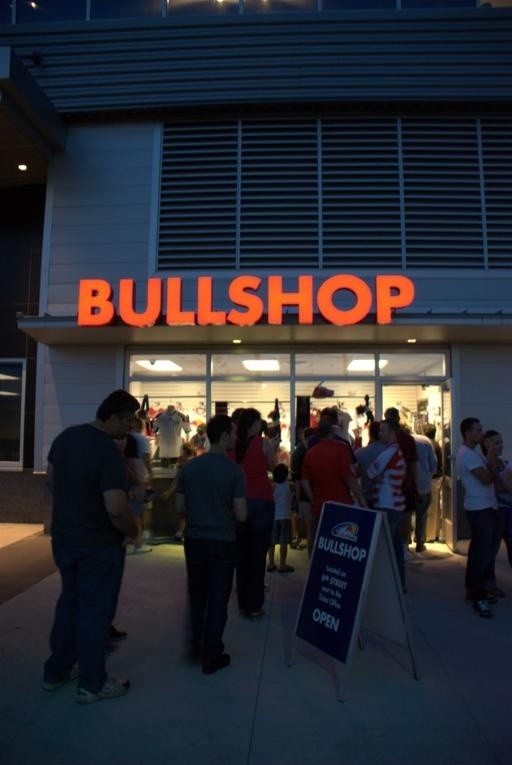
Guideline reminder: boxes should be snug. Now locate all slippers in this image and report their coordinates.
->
[278,566,294,573]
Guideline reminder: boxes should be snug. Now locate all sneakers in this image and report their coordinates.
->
[202,654,230,675]
[476,600,493,617]
[111,626,127,640]
[41,666,79,692]
[133,547,152,554]
[75,677,129,704]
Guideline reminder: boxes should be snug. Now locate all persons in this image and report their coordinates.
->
[106,430,138,656]
[40,389,139,705]
[130,407,511,675]
